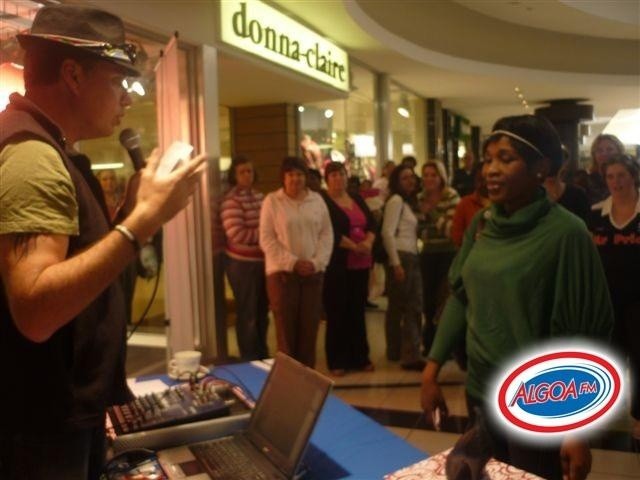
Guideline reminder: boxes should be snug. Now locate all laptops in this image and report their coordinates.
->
[188,349,334,480]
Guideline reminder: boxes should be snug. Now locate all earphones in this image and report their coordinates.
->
[71,63,77,79]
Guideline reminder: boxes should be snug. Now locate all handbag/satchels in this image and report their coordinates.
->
[371,225,390,263]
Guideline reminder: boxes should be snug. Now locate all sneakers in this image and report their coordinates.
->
[363,363,375,371]
[332,369,345,375]
[401,360,426,370]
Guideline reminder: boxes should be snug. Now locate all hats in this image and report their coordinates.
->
[15,6,140,78]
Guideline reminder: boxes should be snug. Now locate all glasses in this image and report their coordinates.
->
[76,42,137,63]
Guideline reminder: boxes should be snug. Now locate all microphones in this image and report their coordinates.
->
[118,127,169,258]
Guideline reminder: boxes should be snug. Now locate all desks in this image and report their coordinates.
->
[127,355,441,480]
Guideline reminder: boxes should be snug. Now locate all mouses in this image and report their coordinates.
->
[96,448,156,474]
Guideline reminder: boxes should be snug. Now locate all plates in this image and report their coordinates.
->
[168,366,212,379]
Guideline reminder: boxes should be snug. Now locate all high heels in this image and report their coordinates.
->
[446,416,493,480]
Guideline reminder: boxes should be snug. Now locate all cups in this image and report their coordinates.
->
[167,349,200,374]
